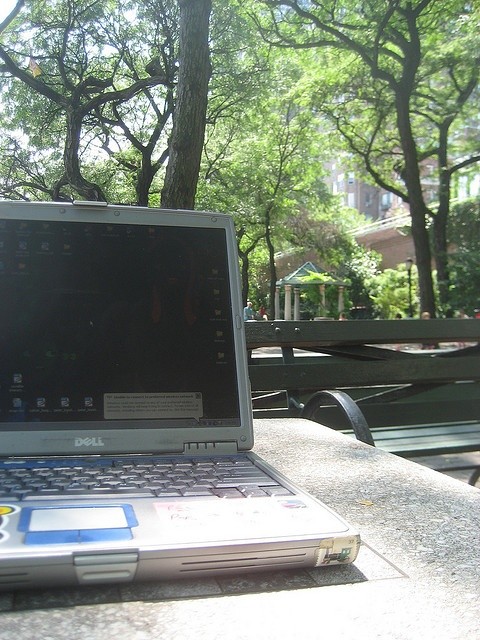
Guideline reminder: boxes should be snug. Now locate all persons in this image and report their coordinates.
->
[339,313,347,320]
[257,305,269,321]
[244,302,255,320]
[459,307,468,348]
[395,313,402,320]
[420,311,436,350]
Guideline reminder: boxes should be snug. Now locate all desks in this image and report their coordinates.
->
[0,419,480,640]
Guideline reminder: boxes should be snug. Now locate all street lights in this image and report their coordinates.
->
[405,257,413,318]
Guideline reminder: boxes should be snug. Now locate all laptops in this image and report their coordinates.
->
[0,199,361,590]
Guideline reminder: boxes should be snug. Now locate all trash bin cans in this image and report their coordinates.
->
[350,306,369,318]
[300,310,313,320]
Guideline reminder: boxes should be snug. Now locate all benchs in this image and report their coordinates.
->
[245,319,480,491]
